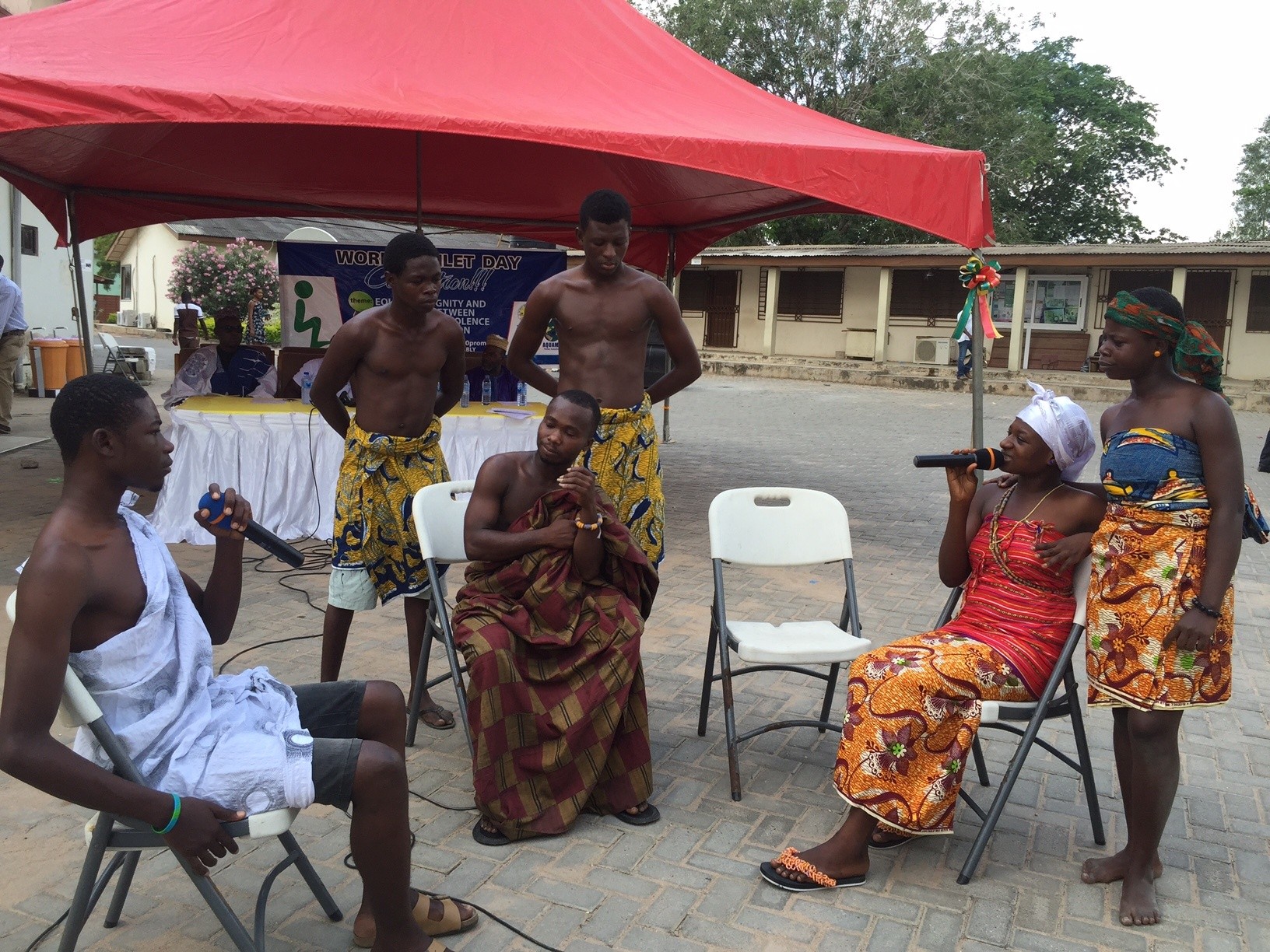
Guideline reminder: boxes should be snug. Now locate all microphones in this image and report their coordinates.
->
[912,447,1003,472]
[198,490,305,569]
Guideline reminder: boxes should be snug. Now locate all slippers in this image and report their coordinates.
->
[760,847,866,892]
[868,825,914,849]
[405,705,456,729]
[352,891,479,948]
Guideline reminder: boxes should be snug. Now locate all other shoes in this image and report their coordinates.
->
[957,375,969,380]
[0,424,11,433]
[615,801,660,825]
[472,814,510,845]
[965,372,972,379]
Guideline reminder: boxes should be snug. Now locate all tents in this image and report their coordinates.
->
[0,0,999,457]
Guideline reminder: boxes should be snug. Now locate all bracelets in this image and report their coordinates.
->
[143,793,182,838]
[574,508,605,530]
[1192,598,1224,619]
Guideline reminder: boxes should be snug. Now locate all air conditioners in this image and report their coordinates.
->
[117,309,153,329]
[913,337,950,366]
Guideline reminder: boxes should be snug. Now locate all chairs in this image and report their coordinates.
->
[897,548,1105,884]
[7,588,343,952]
[99,333,142,386]
[698,487,873,802]
[405,480,476,760]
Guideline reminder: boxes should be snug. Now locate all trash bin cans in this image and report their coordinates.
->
[61,336,88,385]
[26,336,68,390]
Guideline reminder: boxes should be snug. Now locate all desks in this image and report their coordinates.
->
[162,392,548,544]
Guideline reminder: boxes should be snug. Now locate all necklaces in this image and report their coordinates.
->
[988,479,1066,551]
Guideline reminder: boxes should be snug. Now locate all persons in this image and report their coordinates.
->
[309,231,471,730]
[244,287,270,348]
[760,376,1106,895]
[446,387,659,839]
[507,185,702,567]
[1,254,28,435]
[979,288,1246,926]
[0,371,480,952]
[173,291,210,348]
[163,309,276,405]
[282,354,354,404]
[462,332,528,402]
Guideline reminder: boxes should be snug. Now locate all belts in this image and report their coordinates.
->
[2,329,24,338]
